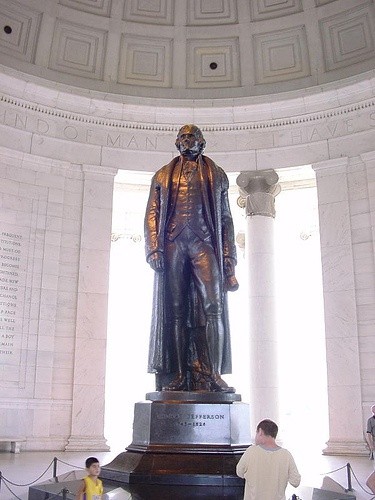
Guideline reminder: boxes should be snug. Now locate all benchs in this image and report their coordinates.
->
[0,435,28,454]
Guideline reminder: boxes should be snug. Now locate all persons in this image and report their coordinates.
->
[77,457,103,500]
[367,406,375,460]
[236,419,300,500]
[145,124,239,393]
